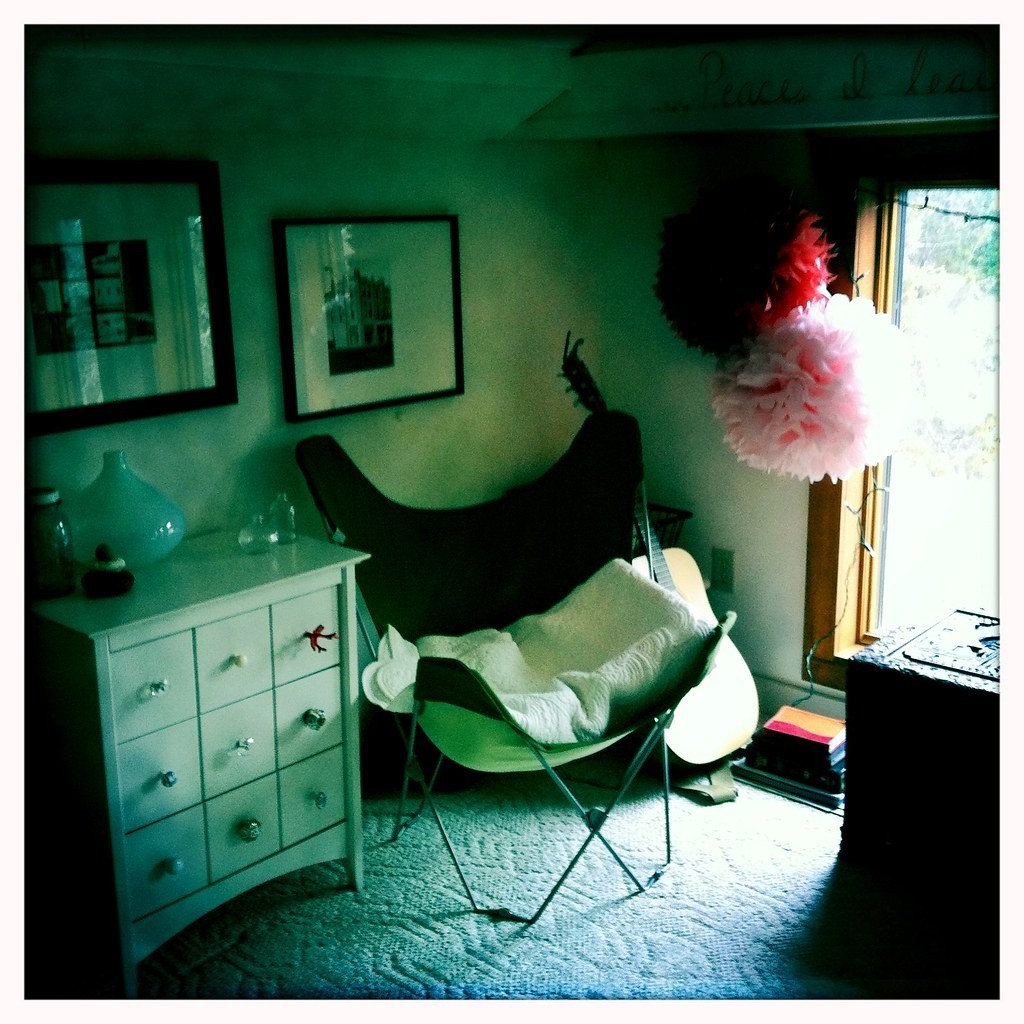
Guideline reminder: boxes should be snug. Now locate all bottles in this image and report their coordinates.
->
[68,449,185,567]
[82,544,135,599]
[238,512,278,555]
[24,488,74,599]
[270,492,296,543]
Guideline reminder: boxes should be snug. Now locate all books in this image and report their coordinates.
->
[732,704,846,808]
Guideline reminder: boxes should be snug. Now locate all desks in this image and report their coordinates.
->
[838,604,1001,913]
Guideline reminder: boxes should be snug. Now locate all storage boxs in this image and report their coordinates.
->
[646,501,695,548]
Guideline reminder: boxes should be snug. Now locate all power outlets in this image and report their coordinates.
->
[710,546,736,596]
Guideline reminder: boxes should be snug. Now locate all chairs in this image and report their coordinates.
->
[292,409,736,925]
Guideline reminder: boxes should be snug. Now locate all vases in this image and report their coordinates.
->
[60,450,192,572]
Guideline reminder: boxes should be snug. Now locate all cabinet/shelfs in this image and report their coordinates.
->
[24,523,376,1000]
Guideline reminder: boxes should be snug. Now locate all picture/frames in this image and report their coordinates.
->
[271,213,468,428]
[25,152,240,448]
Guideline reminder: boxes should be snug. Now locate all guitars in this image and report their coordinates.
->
[629,484,760,765]
